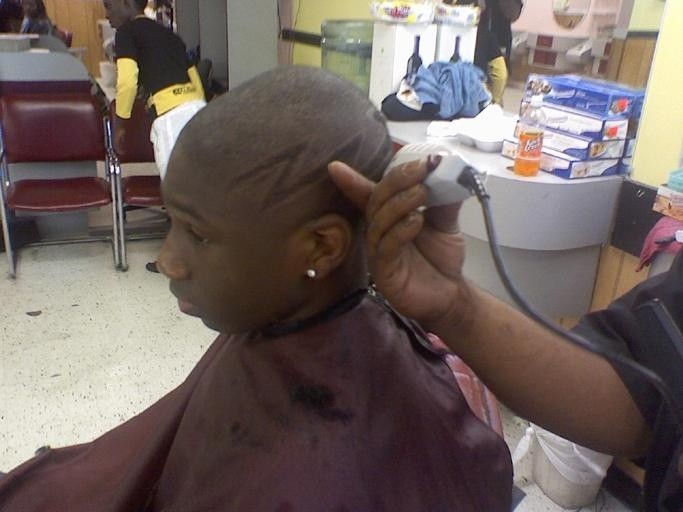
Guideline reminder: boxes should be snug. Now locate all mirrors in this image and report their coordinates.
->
[287,1,668,177]
[0,0,177,123]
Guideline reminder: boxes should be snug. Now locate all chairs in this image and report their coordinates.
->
[107,97,166,272]
[0,93,121,280]
[1,79,95,100]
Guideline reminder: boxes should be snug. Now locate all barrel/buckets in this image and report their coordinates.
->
[322,17,371,97]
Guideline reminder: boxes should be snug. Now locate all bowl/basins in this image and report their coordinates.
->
[369,2,433,25]
[437,4,481,25]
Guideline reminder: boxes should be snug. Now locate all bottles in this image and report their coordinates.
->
[515,94,546,176]
[407,35,423,84]
[450,35,461,63]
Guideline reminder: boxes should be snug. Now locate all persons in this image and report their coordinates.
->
[102,0,211,274]
[0,65,514,511]
[19,1,53,36]
[324,155,683,512]
[442,1,522,111]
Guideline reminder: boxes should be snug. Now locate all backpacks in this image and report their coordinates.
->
[51,24,73,48]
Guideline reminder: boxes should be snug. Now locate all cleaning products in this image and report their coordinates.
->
[516,83,548,176]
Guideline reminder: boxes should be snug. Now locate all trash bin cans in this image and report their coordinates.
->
[529,422,614,510]
